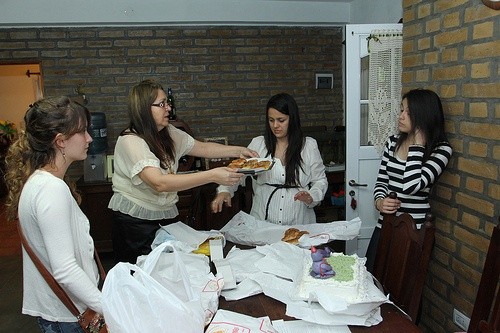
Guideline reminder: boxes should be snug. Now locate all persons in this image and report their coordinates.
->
[212,92,328,225]
[6,96,103,333]
[365,88,454,274]
[108,80,260,263]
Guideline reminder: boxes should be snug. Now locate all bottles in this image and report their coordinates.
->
[87,111,108,155]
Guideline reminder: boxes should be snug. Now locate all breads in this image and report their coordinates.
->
[282,228,309,245]
[229,158,270,170]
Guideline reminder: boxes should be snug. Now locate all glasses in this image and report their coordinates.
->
[151,99,171,107]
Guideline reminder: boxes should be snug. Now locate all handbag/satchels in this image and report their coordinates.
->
[78,306,108,333]
[100,241,219,333]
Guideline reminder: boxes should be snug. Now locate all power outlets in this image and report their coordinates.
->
[453,308,472,331]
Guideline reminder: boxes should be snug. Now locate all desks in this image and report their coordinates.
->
[314,170,345,220]
[217,239,424,333]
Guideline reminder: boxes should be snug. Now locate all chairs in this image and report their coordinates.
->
[378,192,435,325]
[467,215,500,333]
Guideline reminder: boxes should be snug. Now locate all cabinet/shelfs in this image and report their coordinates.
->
[170,121,195,172]
[75,175,252,254]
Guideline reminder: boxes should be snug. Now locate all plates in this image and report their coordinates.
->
[229,159,275,175]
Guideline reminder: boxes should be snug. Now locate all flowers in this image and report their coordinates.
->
[0,119,17,133]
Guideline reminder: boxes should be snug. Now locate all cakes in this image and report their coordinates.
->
[302,247,362,302]
